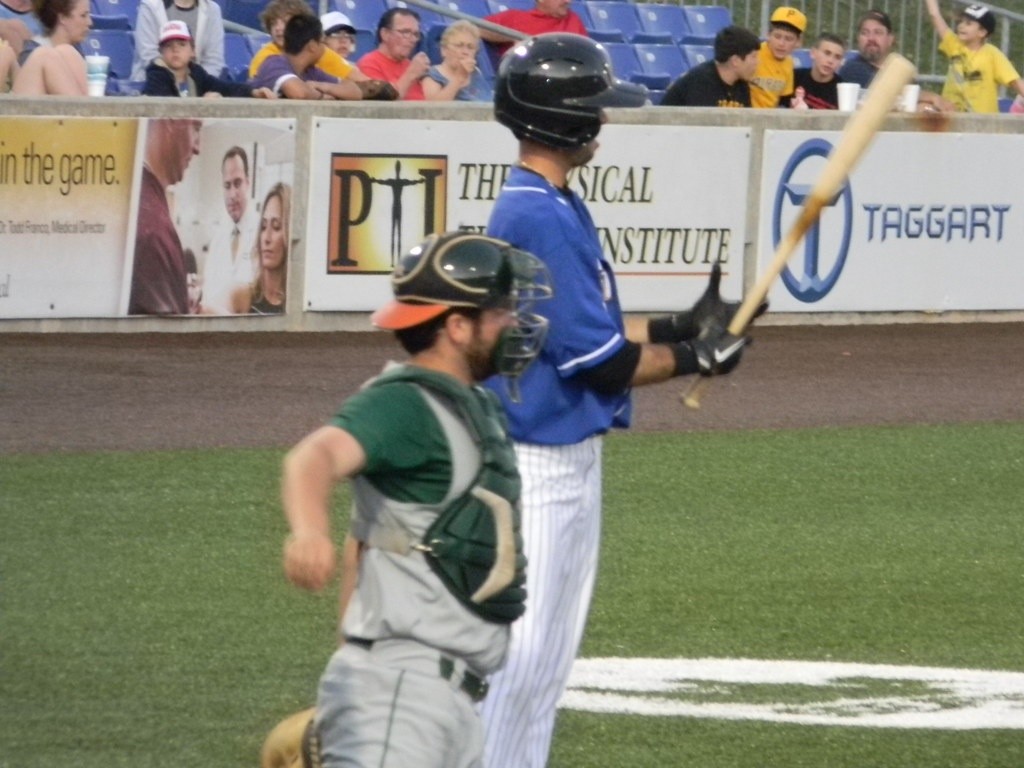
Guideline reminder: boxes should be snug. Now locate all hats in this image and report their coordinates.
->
[771,8,808,33]
[320,10,358,36]
[855,10,891,33]
[959,3,996,32]
[158,19,191,47]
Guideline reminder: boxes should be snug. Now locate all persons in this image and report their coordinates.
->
[197,147,266,314]
[278,231,551,768]
[229,183,285,313]
[128,119,204,316]
[659,0,1024,113]
[132,0,588,101]
[472,31,768,768]
[0,1,91,105]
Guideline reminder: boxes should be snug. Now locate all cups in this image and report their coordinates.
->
[902,83,921,113]
[836,82,861,113]
[85,54,110,98]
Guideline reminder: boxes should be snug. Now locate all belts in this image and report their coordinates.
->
[346,633,488,702]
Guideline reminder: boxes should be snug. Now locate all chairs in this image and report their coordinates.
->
[76,0,862,105]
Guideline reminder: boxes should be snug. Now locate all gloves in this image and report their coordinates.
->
[642,262,770,348]
[670,314,754,380]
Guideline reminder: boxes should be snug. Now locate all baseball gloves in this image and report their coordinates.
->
[261,706,320,767]
[356,78,400,101]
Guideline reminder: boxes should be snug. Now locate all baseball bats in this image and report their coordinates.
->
[676,50,918,412]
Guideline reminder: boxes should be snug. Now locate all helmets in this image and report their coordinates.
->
[493,31,648,153]
[367,232,513,332]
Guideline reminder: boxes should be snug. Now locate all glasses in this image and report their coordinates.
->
[387,26,422,43]
[328,29,354,41]
